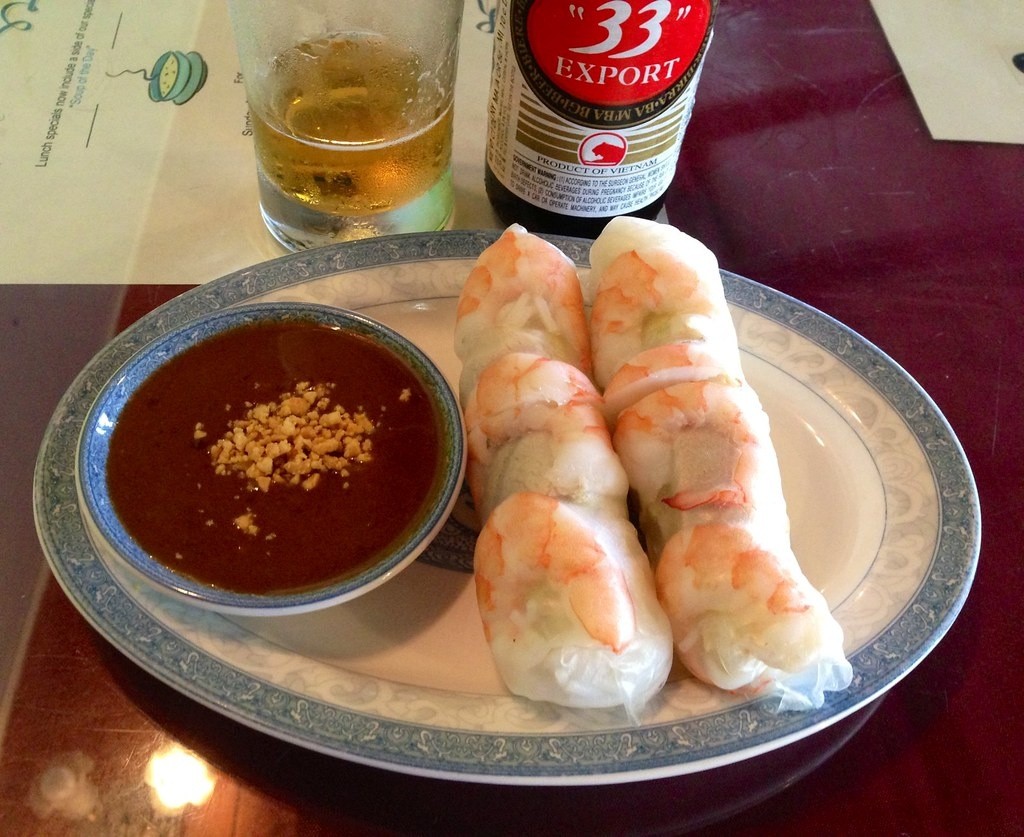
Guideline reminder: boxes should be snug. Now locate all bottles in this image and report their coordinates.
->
[485,0,715,239]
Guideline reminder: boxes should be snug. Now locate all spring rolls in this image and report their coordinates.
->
[451,217,855,722]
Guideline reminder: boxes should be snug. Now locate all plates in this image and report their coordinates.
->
[34,228,981,787]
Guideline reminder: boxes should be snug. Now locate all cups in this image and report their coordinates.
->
[230,0,464,251]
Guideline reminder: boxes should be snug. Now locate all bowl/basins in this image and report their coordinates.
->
[77,300,465,618]
